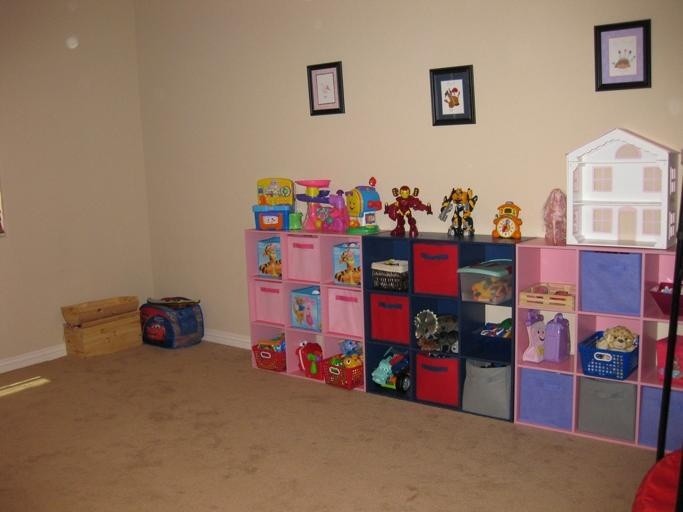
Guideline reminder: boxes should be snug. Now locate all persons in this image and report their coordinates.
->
[437,186,479,238]
[383,185,433,239]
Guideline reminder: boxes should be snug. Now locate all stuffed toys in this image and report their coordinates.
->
[587,325,635,378]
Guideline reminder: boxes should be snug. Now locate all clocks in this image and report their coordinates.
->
[492,201,522,240]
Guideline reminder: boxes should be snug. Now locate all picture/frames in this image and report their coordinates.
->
[430,64,477,126]
[305,61,345,116]
[593,19,654,91]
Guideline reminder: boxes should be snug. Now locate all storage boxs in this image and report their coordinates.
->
[61,296,143,359]
[139,296,205,350]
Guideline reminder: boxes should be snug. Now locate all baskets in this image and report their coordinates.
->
[372,260,408,292]
[320,354,363,390]
[252,344,285,371]
[519,282,576,311]
[579,331,639,381]
